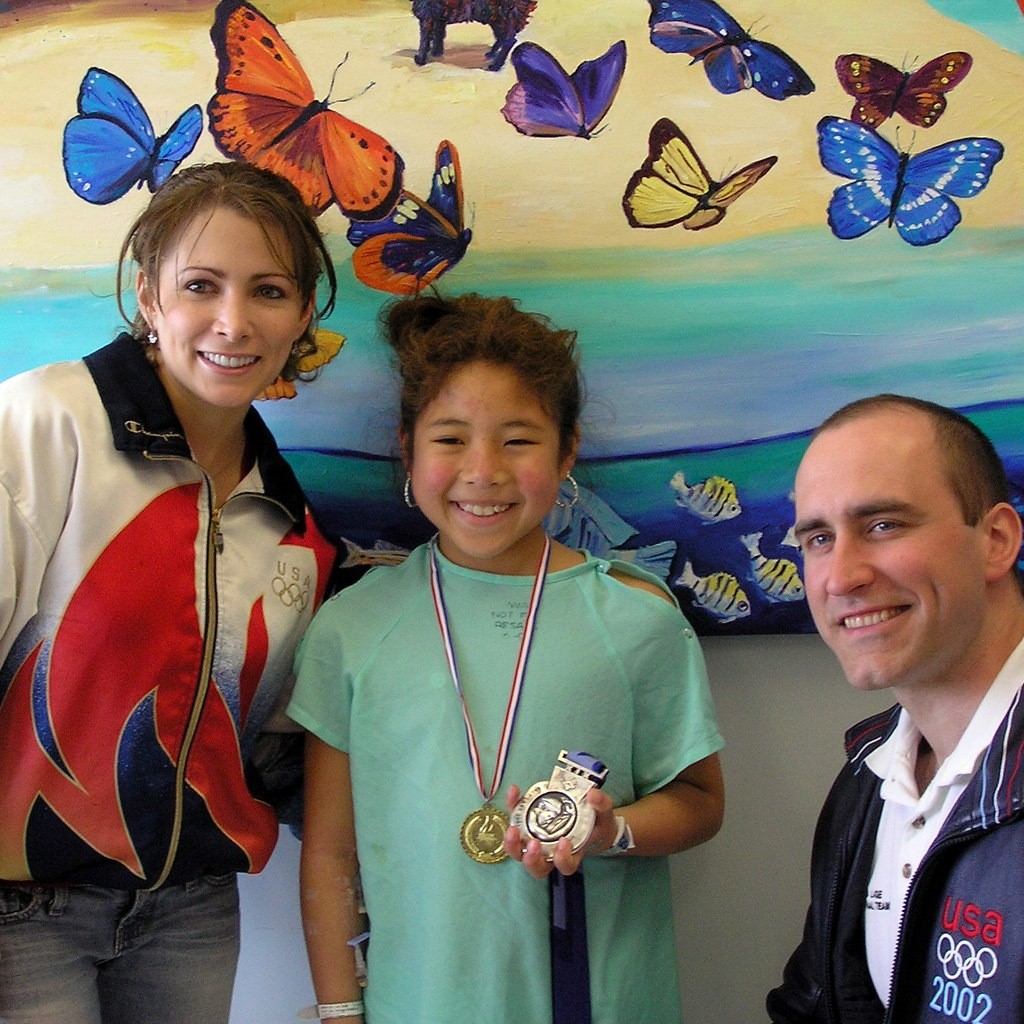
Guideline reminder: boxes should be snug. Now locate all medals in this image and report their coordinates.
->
[460,808,510,863]
[511,751,609,861]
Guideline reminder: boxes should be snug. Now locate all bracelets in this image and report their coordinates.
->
[600,816,635,858]
[316,1001,365,1019]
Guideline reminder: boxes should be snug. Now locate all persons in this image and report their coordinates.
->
[0,163,348,1023]
[766,394,1024,1024]
[285,296,726,1024]
